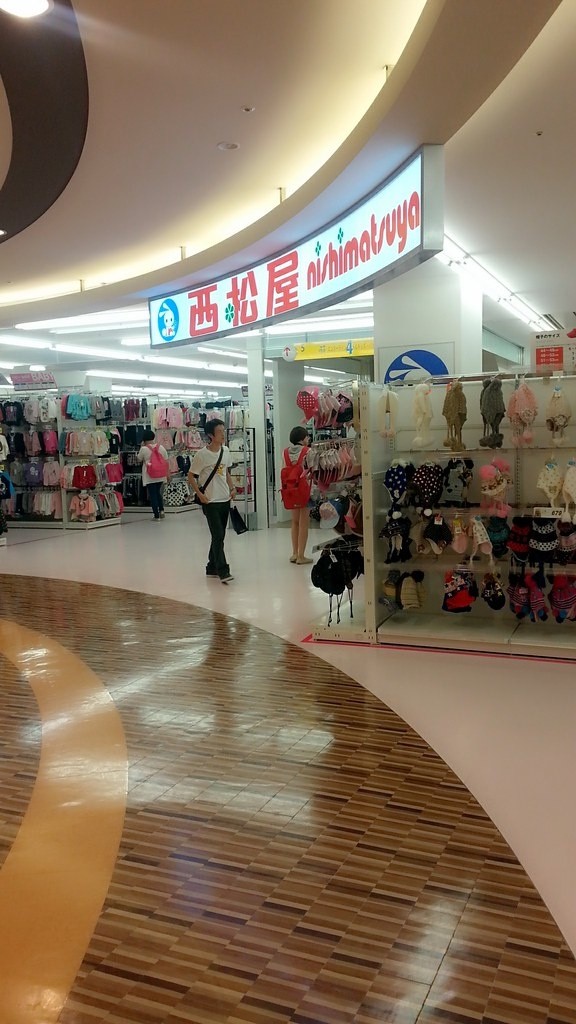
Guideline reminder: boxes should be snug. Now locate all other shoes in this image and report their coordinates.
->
[296,556,313,564]
[218,569,234,583]
[160,511,165,519]
[206,567,220,578]
[151,515,160,521]
[290,553,299,562]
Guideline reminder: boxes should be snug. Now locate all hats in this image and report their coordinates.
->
[365,377,576,625]
[295,388,363,627]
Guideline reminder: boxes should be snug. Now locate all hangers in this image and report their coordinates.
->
[225,405,243,412]
[181,451,188,458]
[2,390,147,495]
[231,434,244,441]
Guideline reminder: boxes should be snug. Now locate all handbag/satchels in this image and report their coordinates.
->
[194,486,206,505]
[229,498,248,535]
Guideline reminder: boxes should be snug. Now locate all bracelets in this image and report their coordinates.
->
[230,486,235,491]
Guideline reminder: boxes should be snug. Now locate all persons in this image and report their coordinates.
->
[281,426,316,564]
[188,418,236,582]
[136,429,170,521]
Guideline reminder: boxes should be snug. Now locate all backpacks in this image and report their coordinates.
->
[147,443,168,478]
[280,447,309,510]
